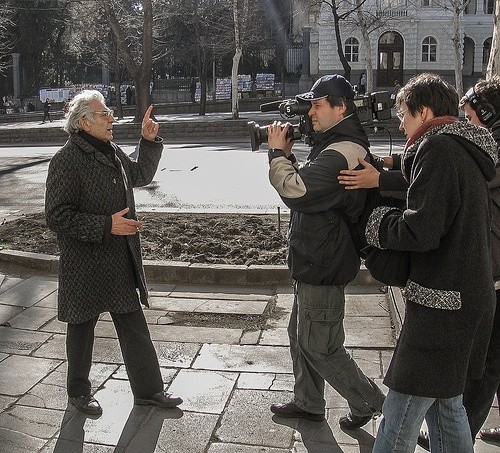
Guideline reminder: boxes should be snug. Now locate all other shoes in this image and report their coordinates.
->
[478,428,500,446]
[416,429,430,451]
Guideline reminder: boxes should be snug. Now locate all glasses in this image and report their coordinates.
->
[396,105,425,122]
[82,110,115,118]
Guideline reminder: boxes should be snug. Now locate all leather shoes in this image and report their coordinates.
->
[134,390,183,408]
[68,393,102,415]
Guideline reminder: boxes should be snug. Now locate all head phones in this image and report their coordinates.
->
[464,87,496,125]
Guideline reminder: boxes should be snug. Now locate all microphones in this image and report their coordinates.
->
[260,101,283,112]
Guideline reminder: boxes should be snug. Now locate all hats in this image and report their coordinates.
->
[296,74,357,101]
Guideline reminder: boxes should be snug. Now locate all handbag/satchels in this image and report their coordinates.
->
[365,247,409,289]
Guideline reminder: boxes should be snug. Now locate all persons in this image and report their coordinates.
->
[393,80,401,95]
[41,98,53,124]
[357,73,366,96]
[337,75,500,453]
[44,89,184,416]
[267,74,386,429]
[356,73,497,453]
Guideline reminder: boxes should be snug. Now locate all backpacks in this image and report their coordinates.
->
[310,137,385,260]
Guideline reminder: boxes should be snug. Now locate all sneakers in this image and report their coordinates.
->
[339,408,383,429]
[270,401,325,422]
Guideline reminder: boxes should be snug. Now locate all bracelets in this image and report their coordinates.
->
[268,148,283,155]
